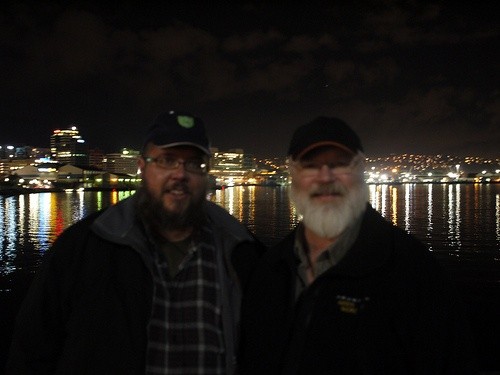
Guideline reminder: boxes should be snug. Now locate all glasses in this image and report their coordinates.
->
[141,153,209,176]
[291,160,361,176]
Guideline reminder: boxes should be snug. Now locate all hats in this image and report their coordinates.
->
[141,108,213,158]
[286,116,363,161]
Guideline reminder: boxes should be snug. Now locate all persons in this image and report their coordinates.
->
[246,115,476,375]
[3,109,268,375]
